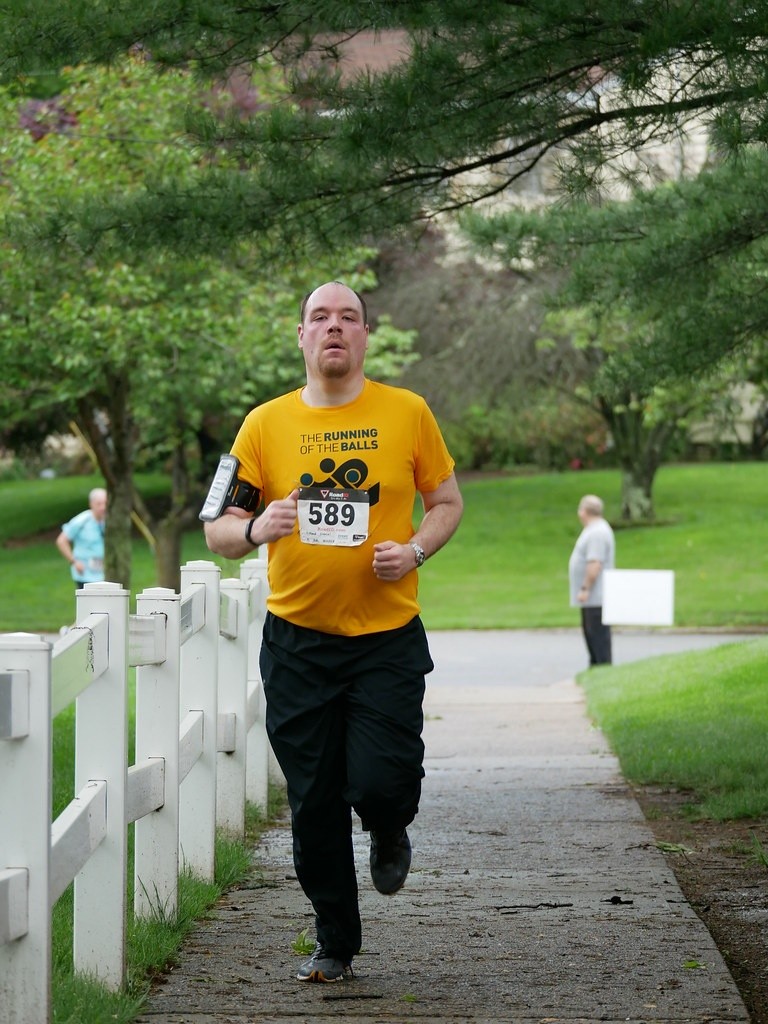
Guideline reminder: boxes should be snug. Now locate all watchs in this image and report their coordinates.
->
[409,540,425,566]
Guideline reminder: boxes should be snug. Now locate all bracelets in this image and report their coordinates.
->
[581,586,590,591]
[244,519,262,546]
[70,559,75,565]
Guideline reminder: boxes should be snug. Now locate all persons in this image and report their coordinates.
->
[202,279,464,983]
[568,493,616,664]
[55,488,107,589]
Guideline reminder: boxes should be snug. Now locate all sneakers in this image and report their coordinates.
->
[369,828,412,895]
[297,942,354,983]
